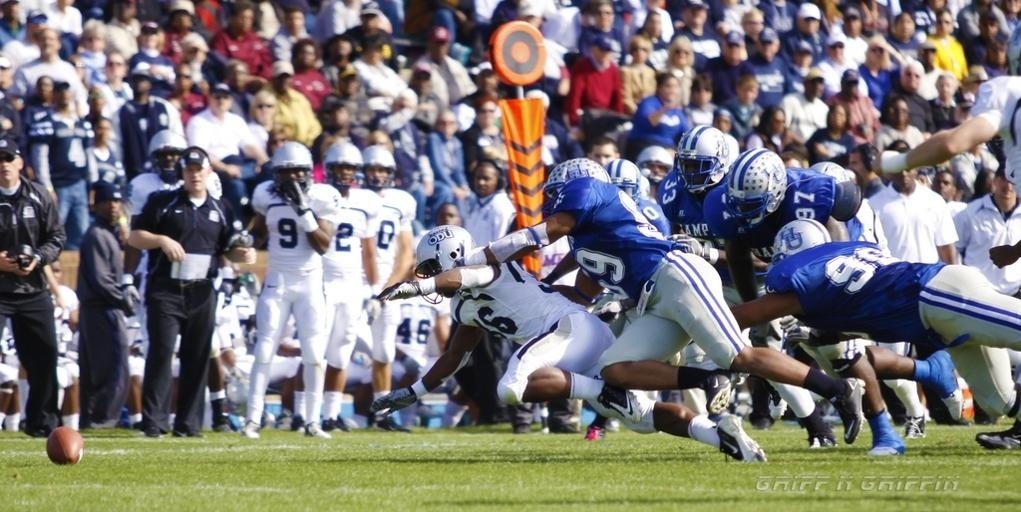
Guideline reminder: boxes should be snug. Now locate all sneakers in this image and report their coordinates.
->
[976,423,1020,449]
[706,350,962,462]
[513,423,581,433]
[584,374,643,440]
[128,410,410,438]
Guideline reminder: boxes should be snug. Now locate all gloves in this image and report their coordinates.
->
[229,231,254,247]
[667,234,710,260]
[284,182,307,215]
[749,322,780,347]
[378,281,422,300]
[121,283,140,318]
[780,315,811,341]
[370,386,417,415]
[218,279,237,308]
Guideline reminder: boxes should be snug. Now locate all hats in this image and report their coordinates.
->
[0,137,17,154]
[95,184,122,201]
[180,147,209,168]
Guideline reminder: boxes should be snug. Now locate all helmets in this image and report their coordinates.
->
[772,218,831,264]
[325,142,364,186]
[272,141,313,187]
[728,146,786,227]
[147,130,187,170]
[605,146,674,204]
[415,225,475,304]
[363,146,396,190]
[675,125,729,193]
[1007,19,1021,76]
[543,159,612,216]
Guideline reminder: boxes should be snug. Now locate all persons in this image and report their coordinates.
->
[2,1,1021,462]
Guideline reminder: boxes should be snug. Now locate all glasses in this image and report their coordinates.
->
[0,154,14,162]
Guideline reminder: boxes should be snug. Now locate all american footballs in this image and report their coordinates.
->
[46,425,84,466]
